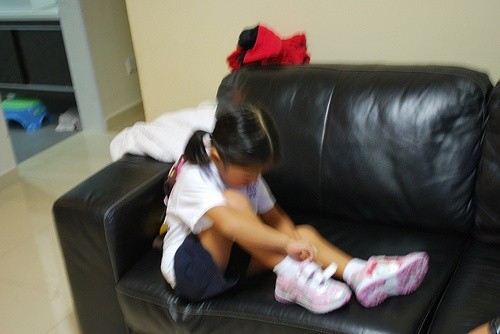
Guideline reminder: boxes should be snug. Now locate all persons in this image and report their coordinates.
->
[161,102,430,313]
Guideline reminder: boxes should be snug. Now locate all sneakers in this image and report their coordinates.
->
[274,258,352,315]
[354,251,428,308]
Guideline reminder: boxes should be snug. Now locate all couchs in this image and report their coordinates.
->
[52,63,500,334]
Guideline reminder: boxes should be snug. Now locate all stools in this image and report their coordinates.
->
[1,99,54,132]
